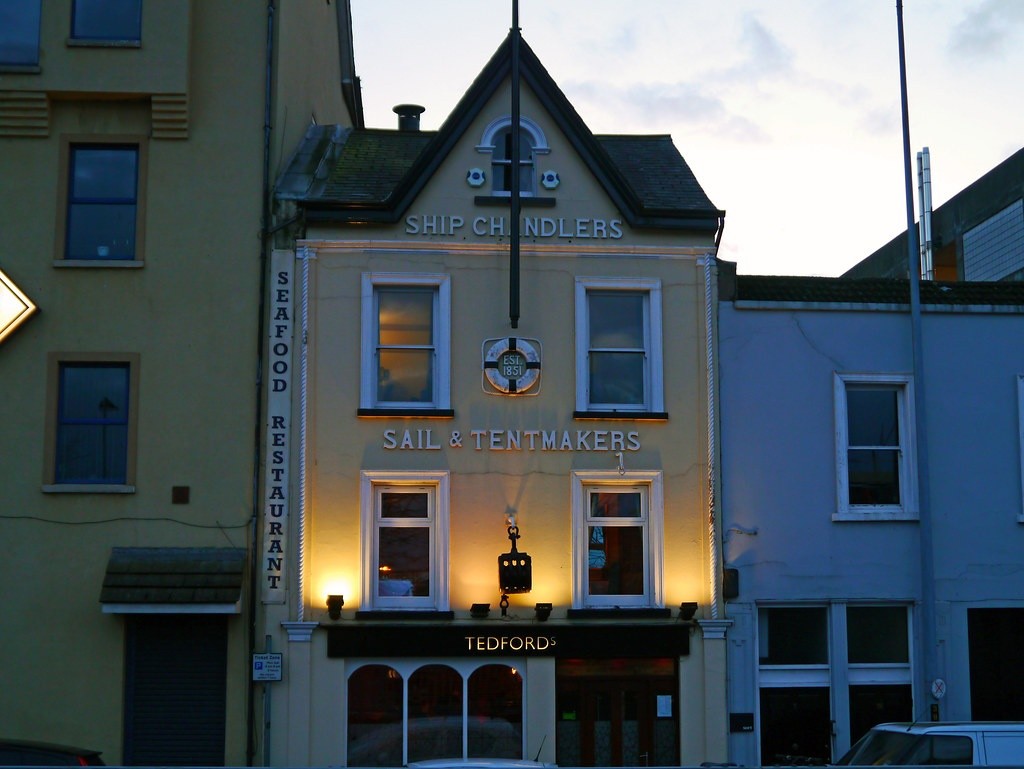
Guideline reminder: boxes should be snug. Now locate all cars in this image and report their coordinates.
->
[406,756,557,768]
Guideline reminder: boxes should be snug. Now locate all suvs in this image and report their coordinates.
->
[1,738,105,766]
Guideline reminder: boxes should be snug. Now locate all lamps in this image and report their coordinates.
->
[679,601,699,621]
[470,603,492,619]
[325,594,345,620]
[535,604,553,620]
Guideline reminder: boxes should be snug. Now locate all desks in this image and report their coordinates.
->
[380,579,416,597]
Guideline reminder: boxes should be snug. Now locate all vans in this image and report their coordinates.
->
[836,718,1024,769]
[347,716,520,766]
